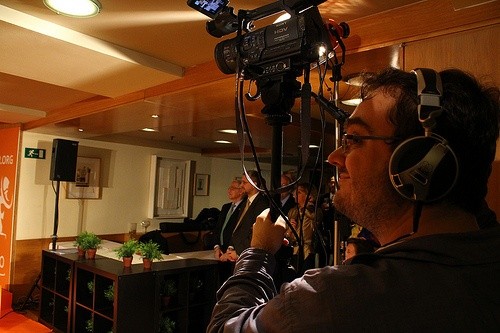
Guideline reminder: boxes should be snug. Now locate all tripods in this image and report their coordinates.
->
[19,181,60,311]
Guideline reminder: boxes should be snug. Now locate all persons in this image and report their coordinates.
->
[205,67,500,333]
[213,168,374,310]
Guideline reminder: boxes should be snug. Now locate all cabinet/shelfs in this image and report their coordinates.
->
[37,249,222,333]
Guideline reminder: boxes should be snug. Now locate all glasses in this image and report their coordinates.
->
[341,133,394,152]
[241,180,249,186]
[228,186,242,191]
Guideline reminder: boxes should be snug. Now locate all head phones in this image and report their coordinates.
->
[388,67,463,203]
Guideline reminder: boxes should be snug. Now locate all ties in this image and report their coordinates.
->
[220,204,236,245]
[233,200,250,234]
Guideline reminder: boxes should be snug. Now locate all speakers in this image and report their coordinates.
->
[49,139,79,182]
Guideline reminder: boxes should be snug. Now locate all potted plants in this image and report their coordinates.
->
[137,239,163,268]
[85,232,102,259]
[77,231,87,257]
[114,238,138,267]
[160,279,177,306]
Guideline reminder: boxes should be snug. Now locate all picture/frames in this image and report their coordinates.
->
[66,157,101,199]
[193,174,208,196]
